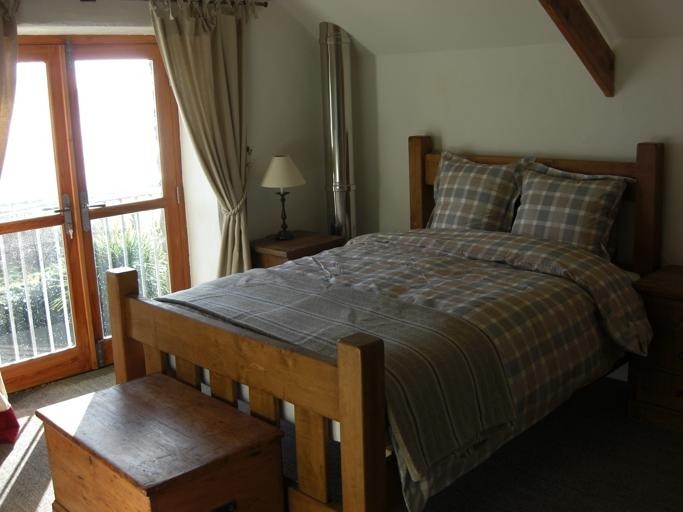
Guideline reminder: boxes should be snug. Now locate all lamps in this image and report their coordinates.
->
[259,154,307,241]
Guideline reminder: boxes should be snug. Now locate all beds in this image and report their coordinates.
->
[106,134,664,511]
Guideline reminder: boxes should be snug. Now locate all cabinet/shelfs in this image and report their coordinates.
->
[35,374,288,512]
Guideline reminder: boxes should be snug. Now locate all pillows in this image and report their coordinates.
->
[509,160,636,260]
[425,151,536,234]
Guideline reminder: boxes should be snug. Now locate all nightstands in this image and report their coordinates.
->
[630,266,682,439]
[250,230,345,268]
